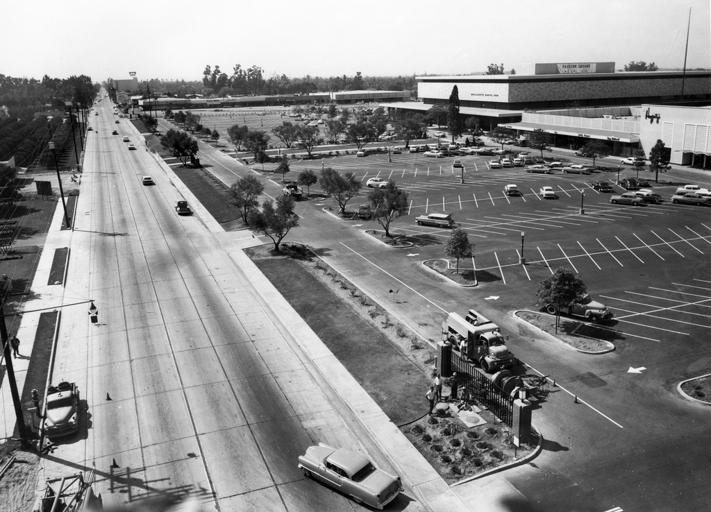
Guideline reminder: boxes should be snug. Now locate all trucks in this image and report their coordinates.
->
[536,293,609,323]
[440,308,515,374]
[44,382,79,435]
[283,184,302,201]
[174,200,193,216]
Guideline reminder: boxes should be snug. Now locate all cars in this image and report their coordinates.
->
[504,184,519,195]
[592,179,613,193]
[671,184,711,205]
[122,136,136,151]
[608,177,662,206]
[539,186,555,199]
[410,143,507,158]
[487,158,590,175]
[356,149,367,157]
[393,147,401,153]
[141,175,153,186]
[453,160,462,167]
[297,441,405,509]
[434,131,445,137]
[366,177,390,189]
[491,136,514,143]
[415,213,454,228]
[620,157,642,166]
[518,151,530,158]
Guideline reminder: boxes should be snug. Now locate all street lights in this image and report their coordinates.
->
[520,231,525,265]
[0,299,99,450]
[580,189,584,214]
[47,98,87,231]
